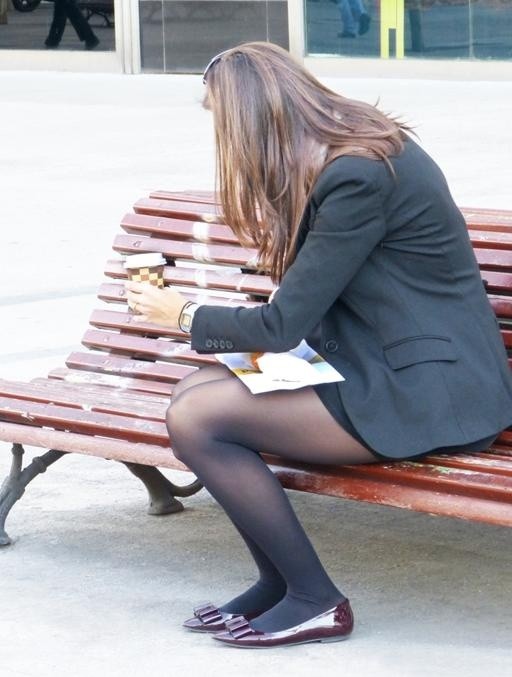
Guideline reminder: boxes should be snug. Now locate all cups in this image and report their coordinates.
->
[123,253,167,288]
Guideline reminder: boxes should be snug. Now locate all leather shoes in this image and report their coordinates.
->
[182,603,247,632]
[212,599,354,649]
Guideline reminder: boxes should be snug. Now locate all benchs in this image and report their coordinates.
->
[0,185,512,547]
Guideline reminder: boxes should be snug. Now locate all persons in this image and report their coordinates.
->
[124,38,511,650]
[43,1,100,50]
[334,0,372,37]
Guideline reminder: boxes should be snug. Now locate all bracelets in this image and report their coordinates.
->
[177,300,196,334]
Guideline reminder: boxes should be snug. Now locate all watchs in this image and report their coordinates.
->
[181,303,202,333]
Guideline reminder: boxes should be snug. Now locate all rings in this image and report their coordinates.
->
[131,303,140,313]
